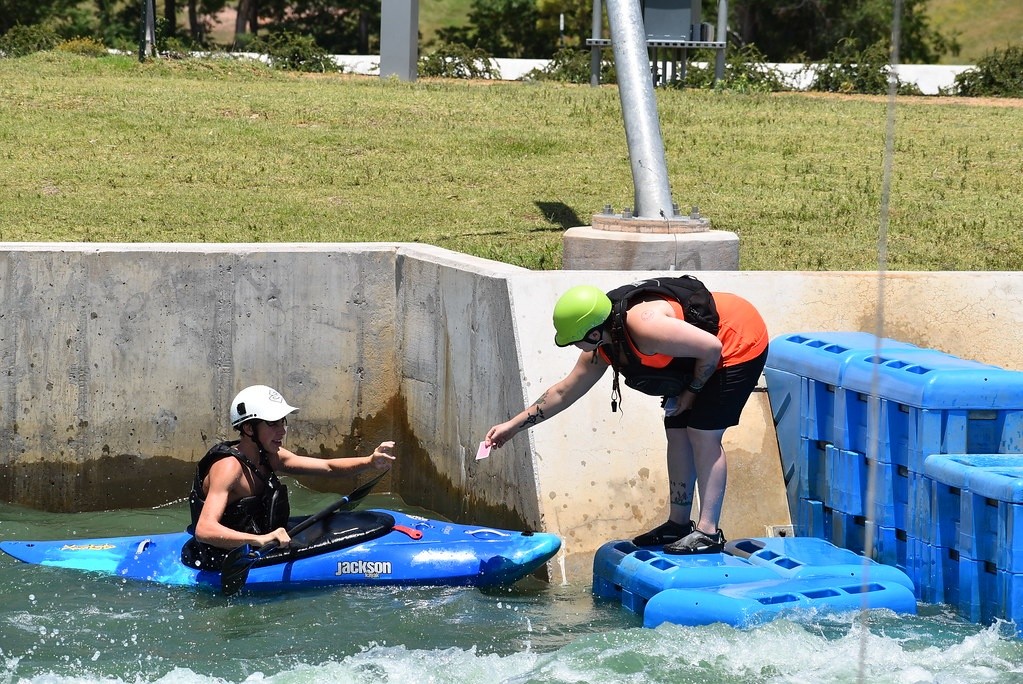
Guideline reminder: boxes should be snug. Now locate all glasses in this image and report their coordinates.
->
[255,416,288,426]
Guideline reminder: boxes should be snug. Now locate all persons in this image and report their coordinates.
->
[484,274,769,554]
[190,384,396,570]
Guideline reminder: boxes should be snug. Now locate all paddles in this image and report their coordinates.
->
[221,467,391,597]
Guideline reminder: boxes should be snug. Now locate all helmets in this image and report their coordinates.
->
[229,385,300,427]
[553,285,613,347]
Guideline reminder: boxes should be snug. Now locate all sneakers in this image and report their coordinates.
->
[632,519,696,549]
[663,529,727,553]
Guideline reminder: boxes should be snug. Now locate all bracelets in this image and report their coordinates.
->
[687,382,704,393]
